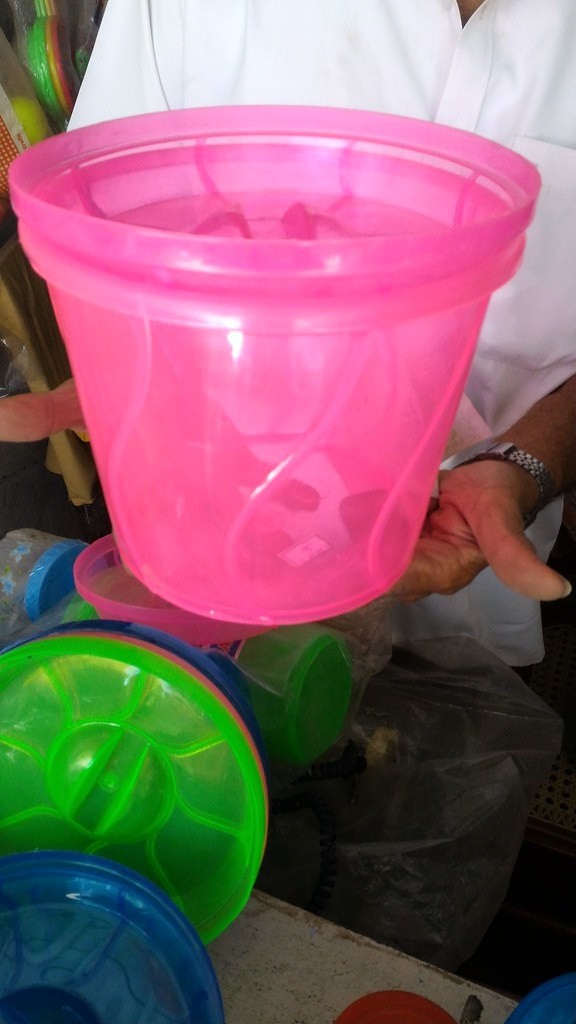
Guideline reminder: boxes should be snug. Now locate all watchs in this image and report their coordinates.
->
[472,440,551,530]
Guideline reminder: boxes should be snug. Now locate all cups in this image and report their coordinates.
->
[9,103,542,624]
[59,577,353,768]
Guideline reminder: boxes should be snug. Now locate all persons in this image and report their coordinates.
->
[59,0,576,688]
